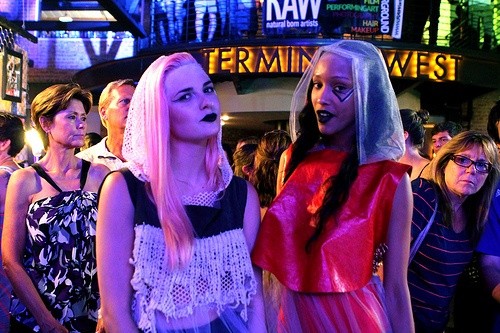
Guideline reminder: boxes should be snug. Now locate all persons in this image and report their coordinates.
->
[7,56,16,96]
[413,0,494,49]
[74,79,139,171]
[80,132,102,153]
[251,40,416,333]
[1,82,111,333]
[222,130,293,333]
[95,51,266,333]
[0,114,25,332]
[399,99,500,333]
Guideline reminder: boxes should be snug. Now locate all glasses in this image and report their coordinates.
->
[449,154,492,173]
